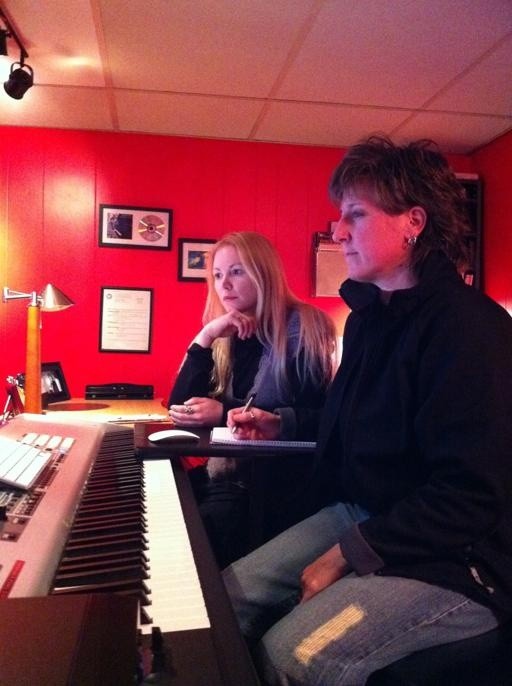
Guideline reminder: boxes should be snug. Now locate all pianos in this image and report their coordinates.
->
[0,413,262,685]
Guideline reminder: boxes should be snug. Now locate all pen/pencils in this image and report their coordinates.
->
[2,395,12,422]
[232,392,257,434]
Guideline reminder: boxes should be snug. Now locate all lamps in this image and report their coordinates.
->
[1,10,35,103]
[0,282,78,418]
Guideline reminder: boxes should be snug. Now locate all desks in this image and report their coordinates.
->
[44,398,215,426]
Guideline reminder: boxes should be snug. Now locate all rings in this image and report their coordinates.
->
[184,405,192,415]
[249,411,255,425]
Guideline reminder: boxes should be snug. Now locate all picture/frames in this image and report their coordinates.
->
[99,203,174,252]
[99,285,154,356]
[177,236,221,285]
[39,360,71,404]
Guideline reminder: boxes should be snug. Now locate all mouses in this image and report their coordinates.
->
[148,429,200,442]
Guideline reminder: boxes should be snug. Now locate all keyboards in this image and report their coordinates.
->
[0,433,53,491]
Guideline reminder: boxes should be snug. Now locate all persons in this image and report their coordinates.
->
[167,233,335,567]
[220,134,512,686]
